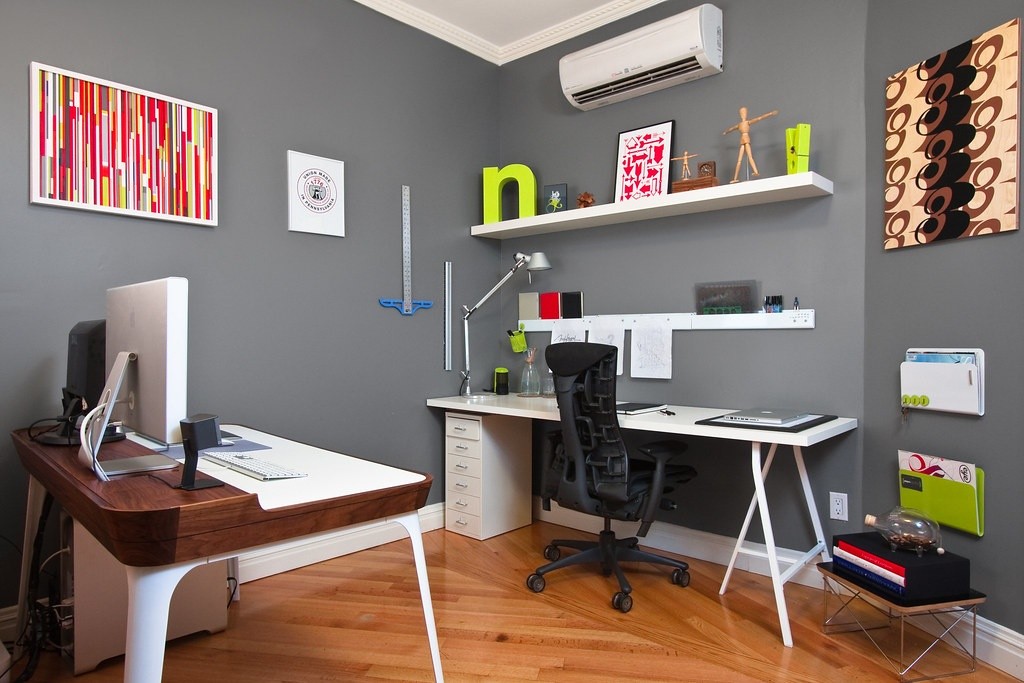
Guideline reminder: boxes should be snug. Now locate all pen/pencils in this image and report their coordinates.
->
[660,409,676,416]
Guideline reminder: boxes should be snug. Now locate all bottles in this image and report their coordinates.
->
[519,347,539,397]
[865,509,944,557]
[543,368,557,397]
[494,367,511,395]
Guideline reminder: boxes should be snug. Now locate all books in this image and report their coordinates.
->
[518,291,583,320]
[832,540,907,595]
[616,402,668,415]
[898,449,977,532]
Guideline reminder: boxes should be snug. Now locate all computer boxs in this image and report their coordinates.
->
[59,510,228,675]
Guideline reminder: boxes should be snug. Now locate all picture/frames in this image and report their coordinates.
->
[29,61,219,227]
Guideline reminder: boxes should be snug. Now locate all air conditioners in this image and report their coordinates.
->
[558,3,723,112]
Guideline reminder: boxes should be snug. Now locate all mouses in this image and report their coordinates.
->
[221,439,235,447]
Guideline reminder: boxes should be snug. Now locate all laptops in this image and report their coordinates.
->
[723,408,809,424]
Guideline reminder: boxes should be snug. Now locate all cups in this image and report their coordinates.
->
[508,330,527,352]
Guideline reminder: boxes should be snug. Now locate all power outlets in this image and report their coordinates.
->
[829,492,849,521]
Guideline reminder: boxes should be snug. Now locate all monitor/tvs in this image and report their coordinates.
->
[38,277,189,476]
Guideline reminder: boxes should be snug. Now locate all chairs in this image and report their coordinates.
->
[527,342,697,612]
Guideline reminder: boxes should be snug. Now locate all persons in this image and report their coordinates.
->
[722,107,777,184]
[671,151,699,178]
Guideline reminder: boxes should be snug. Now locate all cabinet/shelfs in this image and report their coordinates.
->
[445,411,532,541]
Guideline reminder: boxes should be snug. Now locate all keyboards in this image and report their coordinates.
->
[202,452,308,481]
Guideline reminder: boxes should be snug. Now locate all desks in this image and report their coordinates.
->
[8,424,446,683]
[816,562,987,683]
[426,392,858,648]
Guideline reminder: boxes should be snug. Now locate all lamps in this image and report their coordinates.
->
[462,251,553,396]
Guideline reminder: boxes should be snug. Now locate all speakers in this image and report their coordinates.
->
[174,414,225,492]
